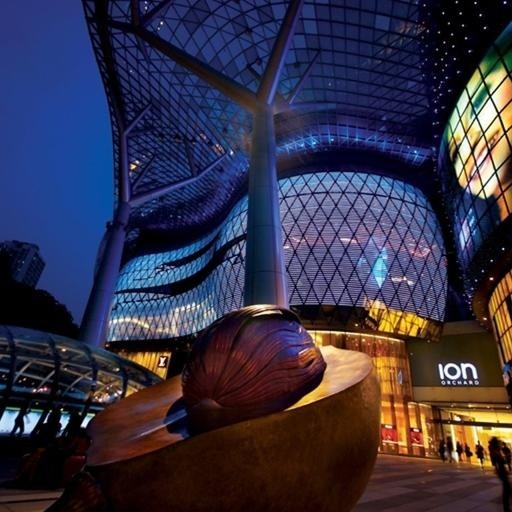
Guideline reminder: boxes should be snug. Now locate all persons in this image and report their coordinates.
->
[61,405,83,438]
[439,31,512,232]
[40,403,62,439]
[488,438,511,511]
[9,401,34,438]
[437,435,512,473]
